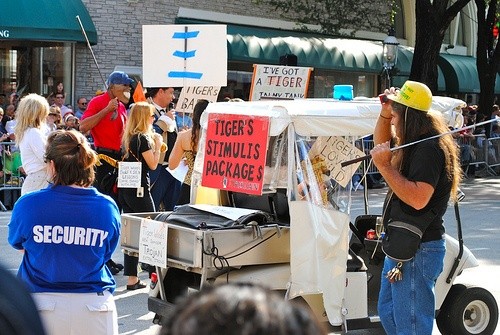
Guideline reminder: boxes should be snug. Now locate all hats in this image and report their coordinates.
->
[385,79,433,113]
[105,71,135,86]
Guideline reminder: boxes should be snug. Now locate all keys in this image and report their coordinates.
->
[386,267,402,283]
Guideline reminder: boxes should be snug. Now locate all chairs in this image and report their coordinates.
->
[270,192,363,273]
[233,191,275,224]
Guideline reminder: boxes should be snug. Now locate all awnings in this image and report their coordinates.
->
[0,0,98,45]
[225,31,500,95]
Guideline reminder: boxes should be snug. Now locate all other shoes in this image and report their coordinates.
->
[109,258,124,271]
[127,280,146,290]
[105,262,119,275]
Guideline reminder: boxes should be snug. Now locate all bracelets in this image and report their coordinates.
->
[380,114,392,120]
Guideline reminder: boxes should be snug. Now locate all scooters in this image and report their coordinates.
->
[121,194,499,335]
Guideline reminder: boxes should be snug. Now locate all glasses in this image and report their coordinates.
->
[149,114,156,118]
[79,103,87,105]
[56,97,63,98]
[48,113,58,116]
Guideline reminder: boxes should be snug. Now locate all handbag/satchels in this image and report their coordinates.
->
[117,160,143,189]
[381,208,437,263]
[99,168,118,195]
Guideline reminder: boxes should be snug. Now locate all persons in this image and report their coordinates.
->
[456,104,500,177]
[146,88,177,137]
[159,283,330,335]
[0,77,96,210]
[80,71,135,277]
[119,102,161,290]
[7,130,122,335]
[167,98,212,211]
[371,80,463,335]
[14,91,54,197]
